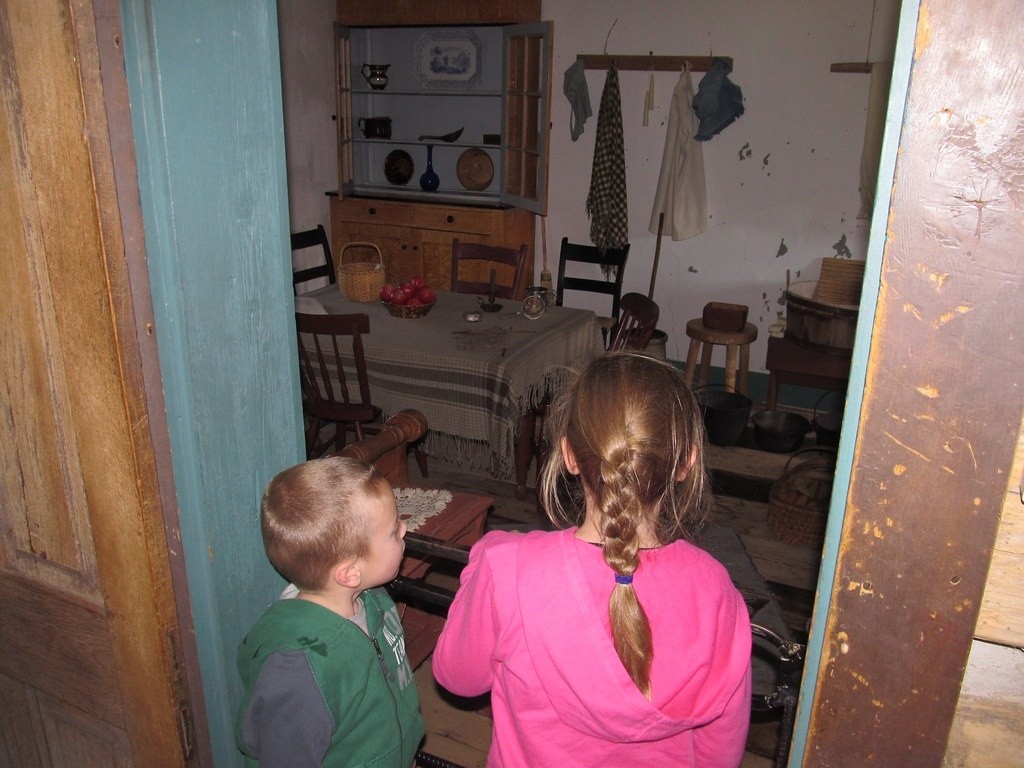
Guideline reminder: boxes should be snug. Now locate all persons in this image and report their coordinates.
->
[234,454,427,767]
[430,348,754,768]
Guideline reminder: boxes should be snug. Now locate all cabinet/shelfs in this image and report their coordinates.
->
[324,0,554,301]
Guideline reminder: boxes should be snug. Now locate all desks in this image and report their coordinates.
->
[295,281,604,501]
[766,331,853,409]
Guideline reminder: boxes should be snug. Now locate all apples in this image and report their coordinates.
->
[380,276,436,306]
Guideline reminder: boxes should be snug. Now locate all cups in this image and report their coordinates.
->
[358,117,393,139]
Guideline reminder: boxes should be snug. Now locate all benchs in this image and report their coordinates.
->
[382,530,804,768]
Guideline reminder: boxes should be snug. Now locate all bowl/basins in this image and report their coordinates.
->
[380,299,436,319]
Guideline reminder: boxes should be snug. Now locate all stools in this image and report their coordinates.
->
[685,318,758,395]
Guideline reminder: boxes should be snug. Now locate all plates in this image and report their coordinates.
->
[457,149,494,190]
[385,149,414,185]
[412,30,482,92]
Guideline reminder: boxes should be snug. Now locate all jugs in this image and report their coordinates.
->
[361,63,392,90]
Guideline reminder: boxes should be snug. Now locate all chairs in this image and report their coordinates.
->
[529,293,659,481]
[290,224,336,296]
[555,237,630,350]
[295,312,430,478]
[450,238,527,301]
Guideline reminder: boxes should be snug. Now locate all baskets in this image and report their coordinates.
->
[768,459,834,551]
[380,298,435,318]
[813,257,866,306]
[338,242,385,302]
